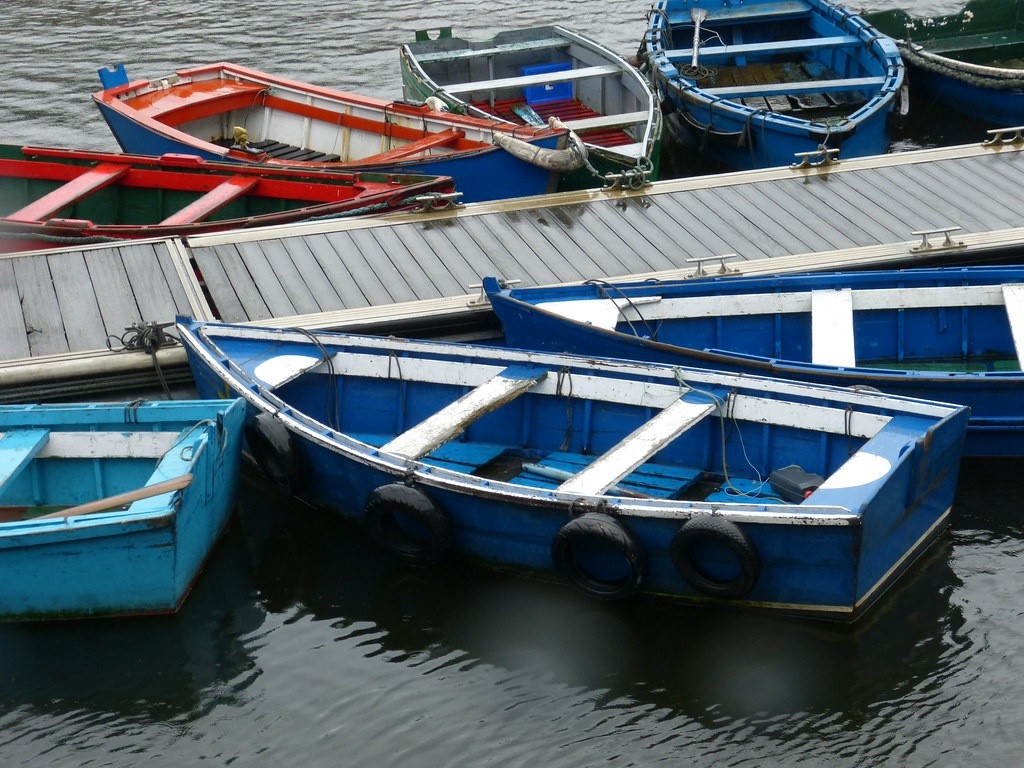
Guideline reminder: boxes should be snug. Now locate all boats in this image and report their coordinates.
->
[482,263,1024,460]
[637,0,908,171]
[0,139,455,254]
[397,24,664,194]
[174,312,972,631]
[92,62,570,206]
[857,0,1024,132]
[0,395,248,626]
